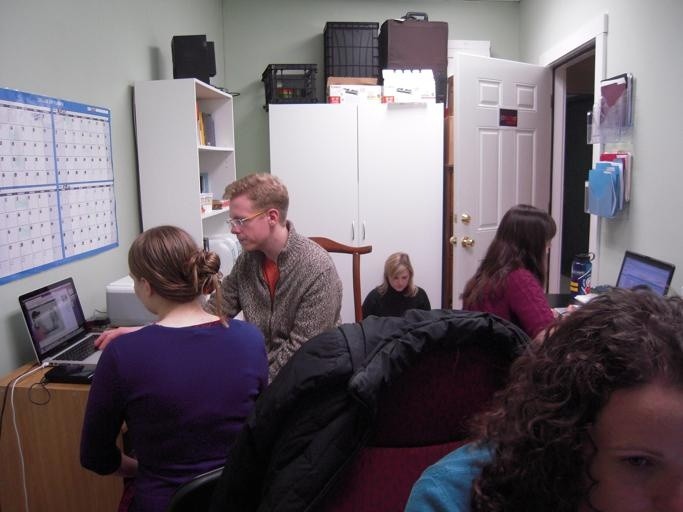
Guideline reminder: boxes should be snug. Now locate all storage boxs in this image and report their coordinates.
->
[323,74,380,102]
[444,39,492,79]
[377,65,438,105]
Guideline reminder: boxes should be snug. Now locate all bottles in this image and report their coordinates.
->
[570,252,595,298]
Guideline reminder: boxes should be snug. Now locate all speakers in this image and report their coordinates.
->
[171,35,216,84]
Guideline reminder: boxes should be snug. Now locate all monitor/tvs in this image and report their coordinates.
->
[574,250,676,306]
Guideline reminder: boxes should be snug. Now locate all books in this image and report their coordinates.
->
[600,151,631,205]
[196,99,216,147]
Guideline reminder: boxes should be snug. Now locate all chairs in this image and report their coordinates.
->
[160,462,227,512]
[296,233,371,332]
[336,341,506,511]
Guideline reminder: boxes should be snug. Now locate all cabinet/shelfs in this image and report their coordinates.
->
[262,100,448,315]
[126,77,244,267]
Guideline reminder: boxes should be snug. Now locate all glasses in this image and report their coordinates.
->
[225,208,269,231]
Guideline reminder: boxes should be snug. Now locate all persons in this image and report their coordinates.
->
[362,252,431,321]
[93,174,343,387]
[79,224,272,511]
[468,283,683,512]
[462,202,560,346]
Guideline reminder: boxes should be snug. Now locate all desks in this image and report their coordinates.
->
[0,357,131,511]
[539,287,577,317]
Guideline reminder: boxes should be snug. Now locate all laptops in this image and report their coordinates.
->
[19,277,117,369]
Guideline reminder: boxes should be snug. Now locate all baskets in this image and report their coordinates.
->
[260,61,321,109]
